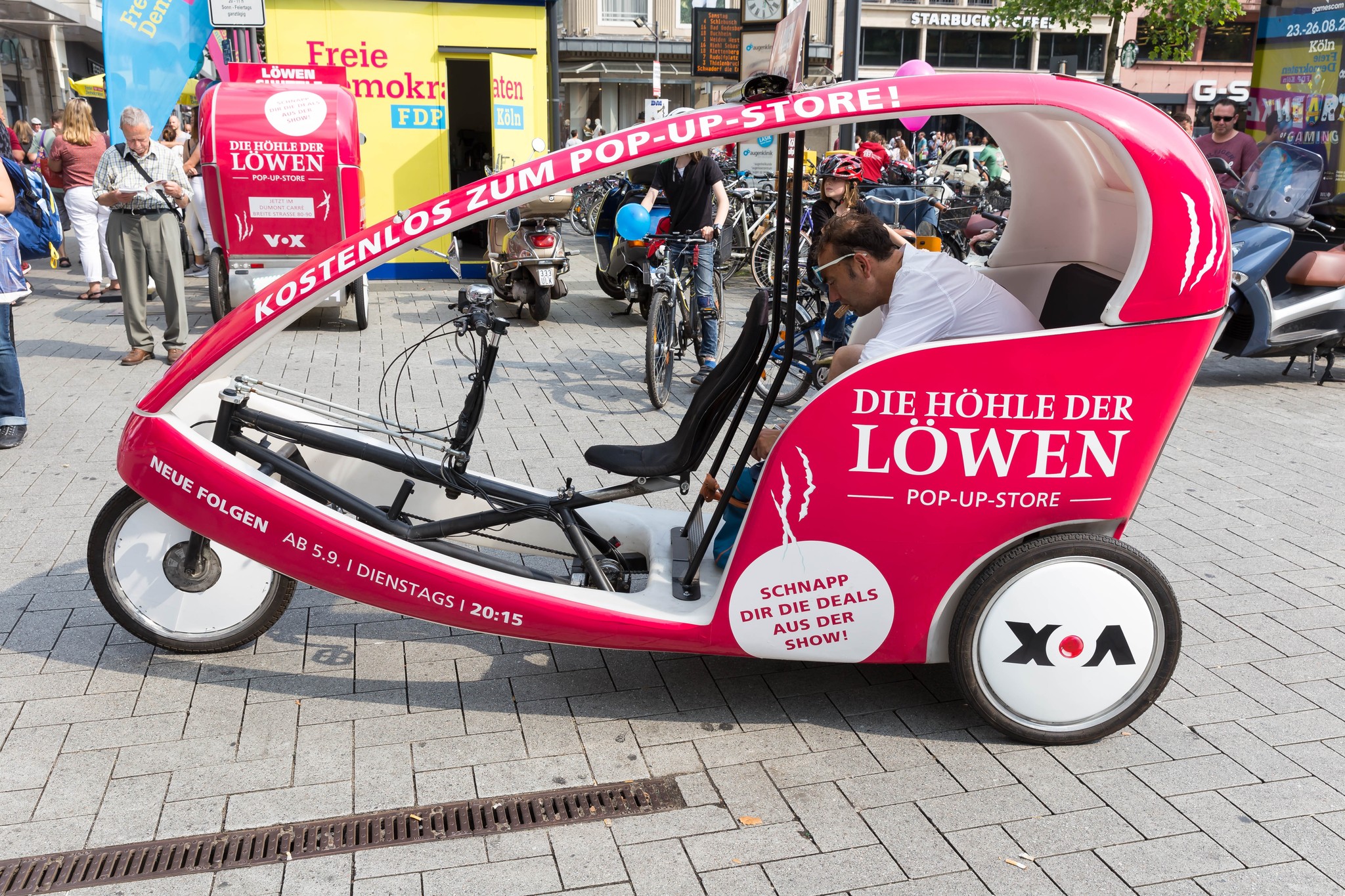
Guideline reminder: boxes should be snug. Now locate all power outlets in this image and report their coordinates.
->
[583,27,589,35]
[561,28,567,35]
[662,29,669,36]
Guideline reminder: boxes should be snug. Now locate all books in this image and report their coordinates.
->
[118,179,168,196]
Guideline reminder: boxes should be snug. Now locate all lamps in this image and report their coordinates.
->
[811,34,818,42]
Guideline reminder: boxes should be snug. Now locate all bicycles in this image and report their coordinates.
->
[568,145,1012,409]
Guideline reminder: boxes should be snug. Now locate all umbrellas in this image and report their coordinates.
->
[68,73,200,107]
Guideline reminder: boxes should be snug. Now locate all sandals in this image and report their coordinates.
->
[100,286,121,295]
[77,290,101,300]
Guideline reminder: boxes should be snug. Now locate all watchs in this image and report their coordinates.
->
[712,223,723,230]
[174,191,185,200]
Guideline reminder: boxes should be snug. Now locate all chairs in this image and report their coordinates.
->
[583,289,770,476]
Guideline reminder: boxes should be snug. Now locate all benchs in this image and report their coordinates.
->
[1038,261,1121,332]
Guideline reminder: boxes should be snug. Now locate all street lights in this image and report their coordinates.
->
[634,17,669,100]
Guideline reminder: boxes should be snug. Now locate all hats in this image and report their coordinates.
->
[930,131,936,137]
[31,118,42,124]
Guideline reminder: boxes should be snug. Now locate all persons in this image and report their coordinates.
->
[641,151,730,384]
[561,119,602,148]
[598,129,606,136]
[93,106,194,365]
[0,96,222,306]
[565,129,583,148]
[0,157,28,448]
[749,100,1259,462]
[1257,110,1292,155]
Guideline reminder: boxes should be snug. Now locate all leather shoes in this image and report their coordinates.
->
[168,348,184,365]
[121,348,155,366]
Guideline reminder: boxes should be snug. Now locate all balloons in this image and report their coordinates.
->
[615,203,650,240]
[893,59,936,131]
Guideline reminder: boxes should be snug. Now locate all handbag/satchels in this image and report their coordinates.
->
[40,159,64,188]
[31,130,45,173]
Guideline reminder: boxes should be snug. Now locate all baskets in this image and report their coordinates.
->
[888,165,915,185]
[775,171,810,200]
[934,179,1010,231]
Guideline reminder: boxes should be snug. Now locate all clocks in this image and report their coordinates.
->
[746,0,781,18]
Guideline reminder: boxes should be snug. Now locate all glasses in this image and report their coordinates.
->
[1212,113,1235,122]
[69,97,87,104]
[812,253,867,283]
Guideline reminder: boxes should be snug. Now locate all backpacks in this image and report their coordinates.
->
[0,156,63,260]
[713,462,766,568]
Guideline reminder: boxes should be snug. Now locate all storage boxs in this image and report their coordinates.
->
[626,161,661,186]
[527,187,576,213]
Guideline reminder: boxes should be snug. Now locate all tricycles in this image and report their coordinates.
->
[85,58,1233,746]
[196,61,370,331]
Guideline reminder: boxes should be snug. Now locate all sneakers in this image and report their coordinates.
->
[815,344,835,364]
[0,425,28,448]
[690,365,714,385]
[21,261,31,274]
[644,358,665,382]
[184,262,210,276]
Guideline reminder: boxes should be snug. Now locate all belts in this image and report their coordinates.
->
[188,173,202,178]
[112,208,171,216]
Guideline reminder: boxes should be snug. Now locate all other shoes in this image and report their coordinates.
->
[59,257,71,267]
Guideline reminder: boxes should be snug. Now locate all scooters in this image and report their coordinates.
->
[1208,141,1345,387]
[480,165,574,322]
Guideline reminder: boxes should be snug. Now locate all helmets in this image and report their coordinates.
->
[817,154,863,183]
[666,107,695,117]
